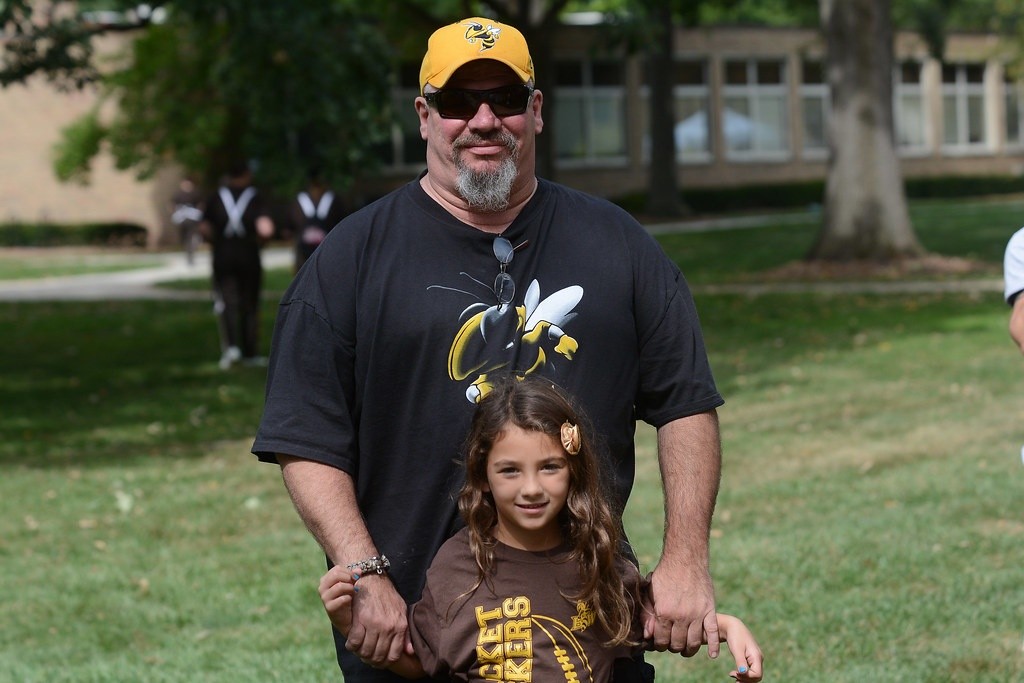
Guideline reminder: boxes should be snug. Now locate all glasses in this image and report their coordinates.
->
[493,233,528,311]
[424,85,529,119]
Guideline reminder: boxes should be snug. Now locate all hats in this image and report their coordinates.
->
[419,17,535,97]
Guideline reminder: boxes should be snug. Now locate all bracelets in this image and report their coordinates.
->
[347,555,390,575]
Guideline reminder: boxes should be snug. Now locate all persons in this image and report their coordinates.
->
[174,200,201,262]
[318,380,764,683]
[291,163,353,268]
[198,158,276,371]
[250,17,724,683]
